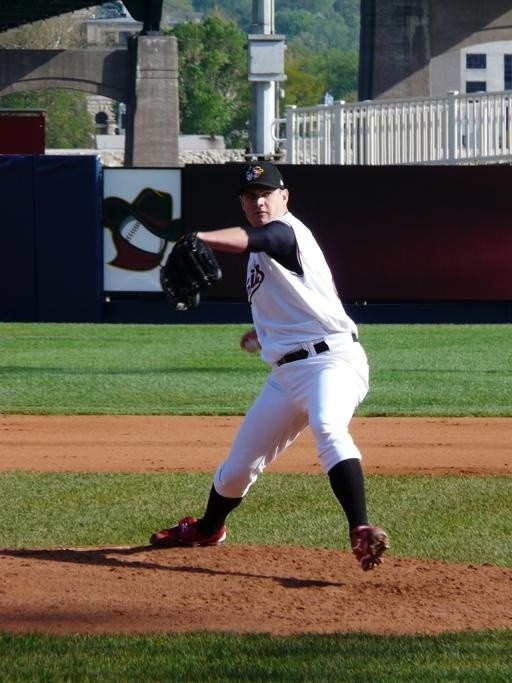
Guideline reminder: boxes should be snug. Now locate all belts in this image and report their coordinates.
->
[276,332,358,366]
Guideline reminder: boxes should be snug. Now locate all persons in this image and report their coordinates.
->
[149,159,389,571]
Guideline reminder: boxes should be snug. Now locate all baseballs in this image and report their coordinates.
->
[245,338,258,352]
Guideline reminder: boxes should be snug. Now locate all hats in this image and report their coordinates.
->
[103,187,181,242]
[239,161,284,193]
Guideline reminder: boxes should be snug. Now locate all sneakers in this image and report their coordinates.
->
[349,523,390,571]
[149,515,227,546]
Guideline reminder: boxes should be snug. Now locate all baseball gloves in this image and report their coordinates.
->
[159,233,224,314]
